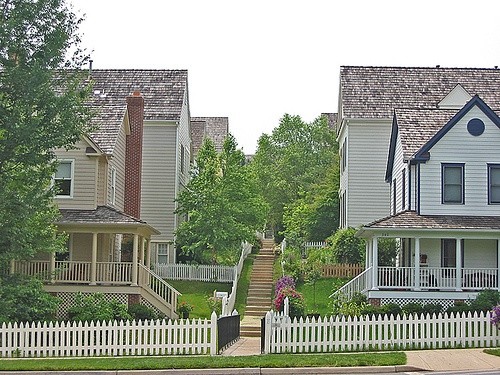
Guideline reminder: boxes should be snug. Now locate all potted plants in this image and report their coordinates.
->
[421,254,427,263]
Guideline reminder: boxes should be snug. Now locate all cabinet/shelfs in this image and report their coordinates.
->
[418,263,429,292]
